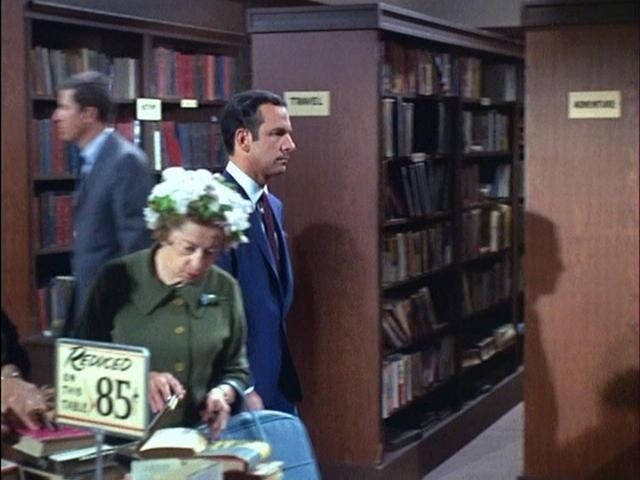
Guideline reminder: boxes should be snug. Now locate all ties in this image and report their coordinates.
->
[261,192,277,259]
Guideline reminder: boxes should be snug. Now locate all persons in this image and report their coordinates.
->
[67,167,252,446]
[208,87,304,421]
[48,69,158,339]
[0,305,59,433]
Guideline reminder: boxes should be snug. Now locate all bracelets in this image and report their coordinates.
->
[214,384,231,406]
[1,371,23,381]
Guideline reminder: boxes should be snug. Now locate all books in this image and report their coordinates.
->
[79,44,243,177]
[192,437,273,474]
[222,459,285,479]
[133,386,209,460]
[127,454,223,480]
[382,41,525,450]
[25,43,79,337]
[1,425,140,480]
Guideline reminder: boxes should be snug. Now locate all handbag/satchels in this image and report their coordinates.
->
[193,379,319,479]
